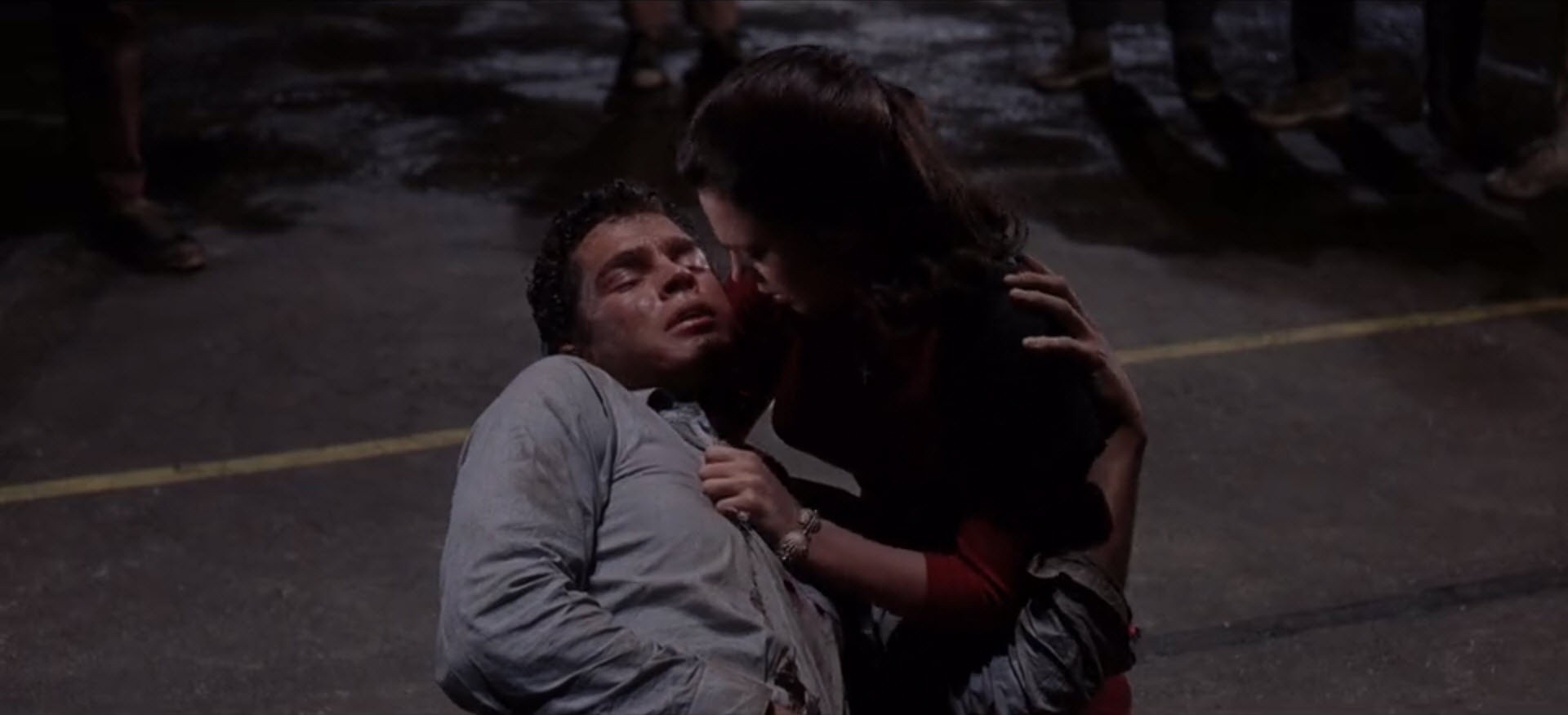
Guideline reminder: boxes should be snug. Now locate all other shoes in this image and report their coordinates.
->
[100,200,205,269]
[1172,49,1221,99]
[622,36,667,90]
[1485,139,1568,199]
[1425,96,1481,160]
[701,33,742,77]
[1024,50,1114,91]
[1249,79,1351,123]
[208,181,298,231]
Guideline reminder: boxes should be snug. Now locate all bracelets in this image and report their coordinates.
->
[780,508,822,562]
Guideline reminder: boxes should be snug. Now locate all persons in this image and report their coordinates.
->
[435,182,1148,715]
[0,0,205,271]
[678,44,1136,715]
[1483,55,1568,201]
[1251,0,1487,167]
[535,0,749,218]
[1042,0,1221,100]
